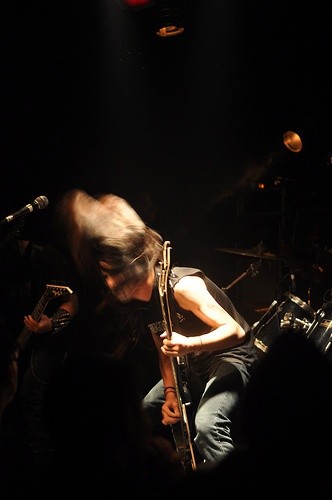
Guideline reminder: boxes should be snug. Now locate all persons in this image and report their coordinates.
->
[0,191,260,467]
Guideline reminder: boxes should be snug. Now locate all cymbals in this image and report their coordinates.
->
[211,245,287,262]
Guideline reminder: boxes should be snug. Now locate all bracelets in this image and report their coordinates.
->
[163,387,176,397]
[49,309,72,335]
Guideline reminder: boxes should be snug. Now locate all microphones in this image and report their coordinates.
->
[2,196,49,224]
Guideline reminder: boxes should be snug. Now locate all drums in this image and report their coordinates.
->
[247,288,318,353]
[305,317,332,354]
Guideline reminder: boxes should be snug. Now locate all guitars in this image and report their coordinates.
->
[0,283,75,417]
[158,241,205,472]
